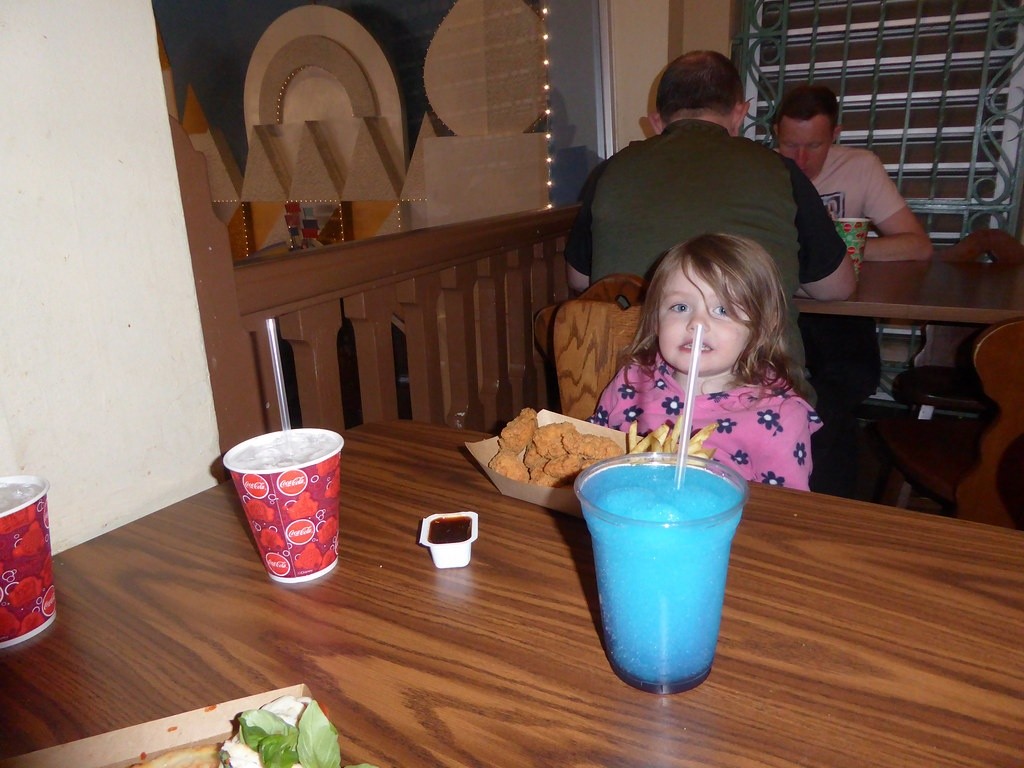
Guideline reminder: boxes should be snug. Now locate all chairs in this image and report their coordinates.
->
[872,317,1024,528]
[530,271,651,414]
[904,222,1024,417]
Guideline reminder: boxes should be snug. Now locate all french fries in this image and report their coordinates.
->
[627,414,719,461]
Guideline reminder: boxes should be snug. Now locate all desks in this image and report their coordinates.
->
[771,256,1024,328]
[0,412,1024,768]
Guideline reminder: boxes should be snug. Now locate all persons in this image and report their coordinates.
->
[562,50,857,306]
[766,84,934,500]
[585,231,825,493]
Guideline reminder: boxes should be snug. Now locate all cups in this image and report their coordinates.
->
[832,217,871,281]
[223,427,345,584]
[0,473,58,650]
[573,451,750,695]
[419,511,479,569]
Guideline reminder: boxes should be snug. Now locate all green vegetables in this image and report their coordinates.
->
[235,698,379,768]
